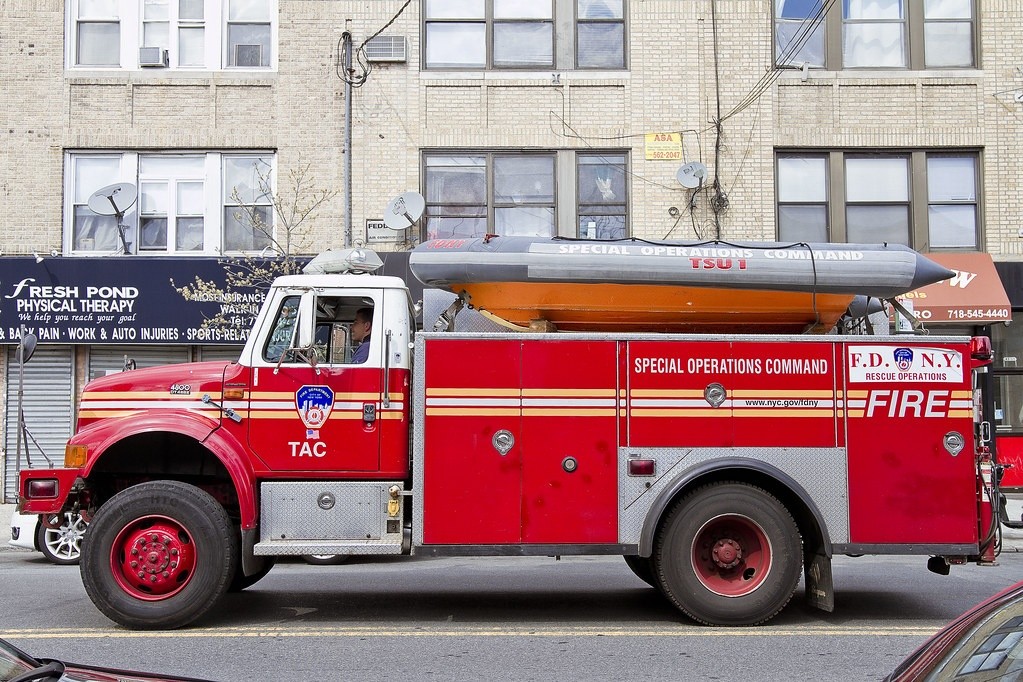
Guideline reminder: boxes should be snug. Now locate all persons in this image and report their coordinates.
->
[350,308,374,364]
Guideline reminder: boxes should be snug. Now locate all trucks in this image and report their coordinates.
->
[18,228,1001,644]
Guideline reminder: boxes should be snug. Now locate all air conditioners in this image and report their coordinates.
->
[138,46,167,67]
[365,34,408,63]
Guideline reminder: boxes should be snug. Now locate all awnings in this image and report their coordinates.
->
[888,251,1012,325]
